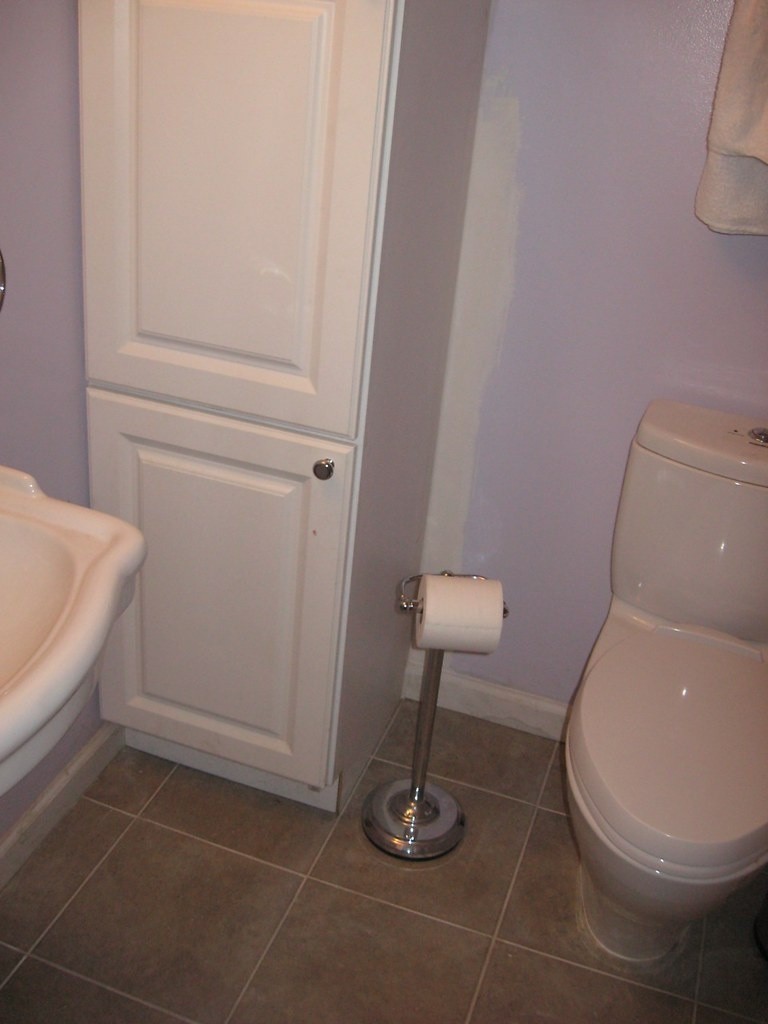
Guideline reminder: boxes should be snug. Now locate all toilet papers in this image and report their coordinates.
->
[414,573,504,656]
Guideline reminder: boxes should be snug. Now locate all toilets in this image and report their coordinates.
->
[564,399,768,963]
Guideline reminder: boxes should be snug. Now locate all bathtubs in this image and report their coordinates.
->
[0,463,147,890]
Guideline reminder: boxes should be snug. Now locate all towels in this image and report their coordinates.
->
[693,0,768,239]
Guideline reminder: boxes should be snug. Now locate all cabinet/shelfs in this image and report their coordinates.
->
[74,0,495,815]
